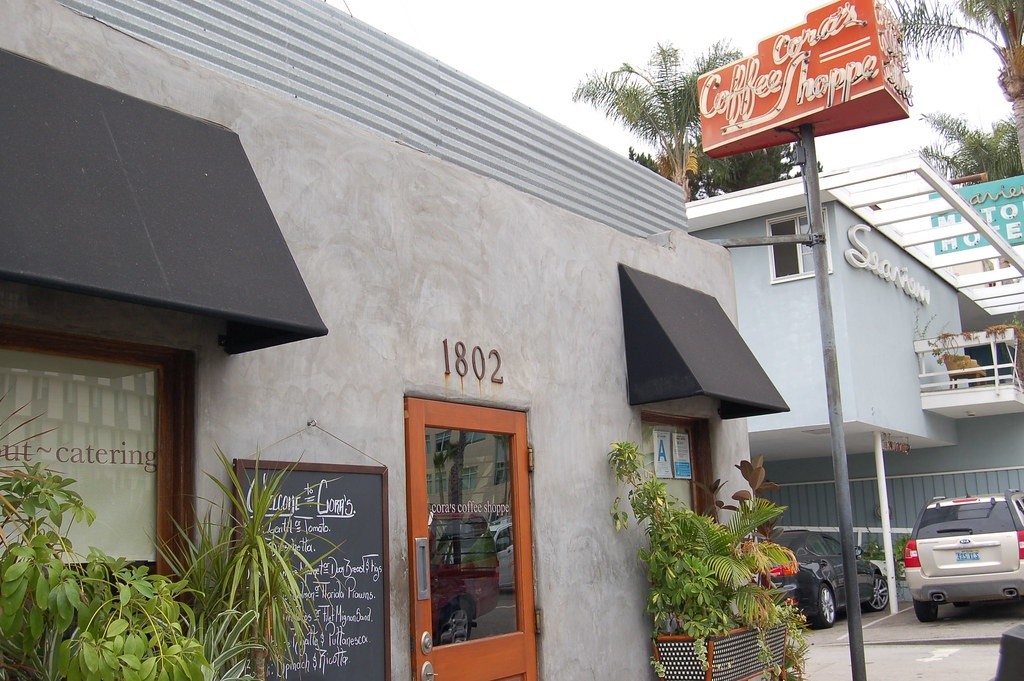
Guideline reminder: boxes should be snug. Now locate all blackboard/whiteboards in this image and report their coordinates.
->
[233,457,392,681]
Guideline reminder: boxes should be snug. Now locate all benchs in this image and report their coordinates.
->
[943,355,987,389]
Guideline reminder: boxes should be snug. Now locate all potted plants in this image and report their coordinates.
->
[605,442,814,681]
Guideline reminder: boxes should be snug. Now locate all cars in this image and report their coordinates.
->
[445,513,514,590]
[753,529,889,630]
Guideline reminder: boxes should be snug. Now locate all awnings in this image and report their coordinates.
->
[619,264,790,420]
[0,49,328,354]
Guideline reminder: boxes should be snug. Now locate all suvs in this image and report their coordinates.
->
[428,512,500,647]
[904,487,1024,623]
